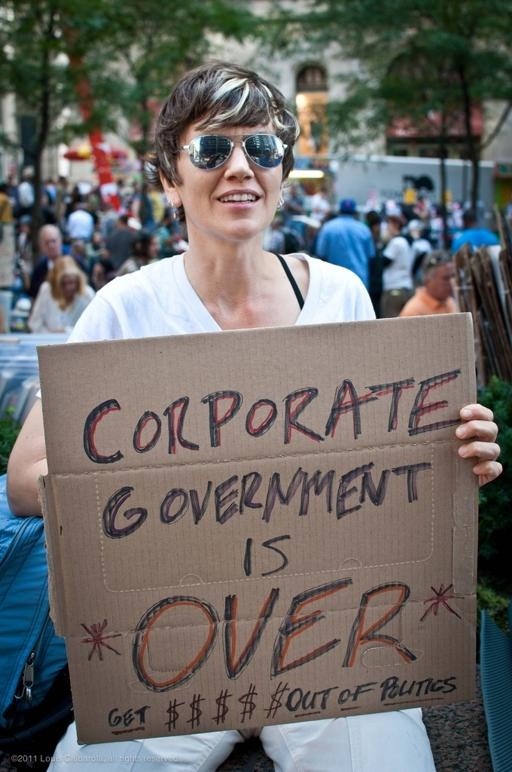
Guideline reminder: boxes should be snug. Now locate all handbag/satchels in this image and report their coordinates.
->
[0,475,66,726]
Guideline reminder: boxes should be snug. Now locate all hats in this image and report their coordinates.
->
[341,200,355,212]
[410,220,422,232]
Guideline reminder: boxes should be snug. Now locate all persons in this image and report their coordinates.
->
[4,63,501,770]
[265,173,504,319]
[0,163,188,335]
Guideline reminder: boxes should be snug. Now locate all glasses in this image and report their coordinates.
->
[172,133,288,170]
